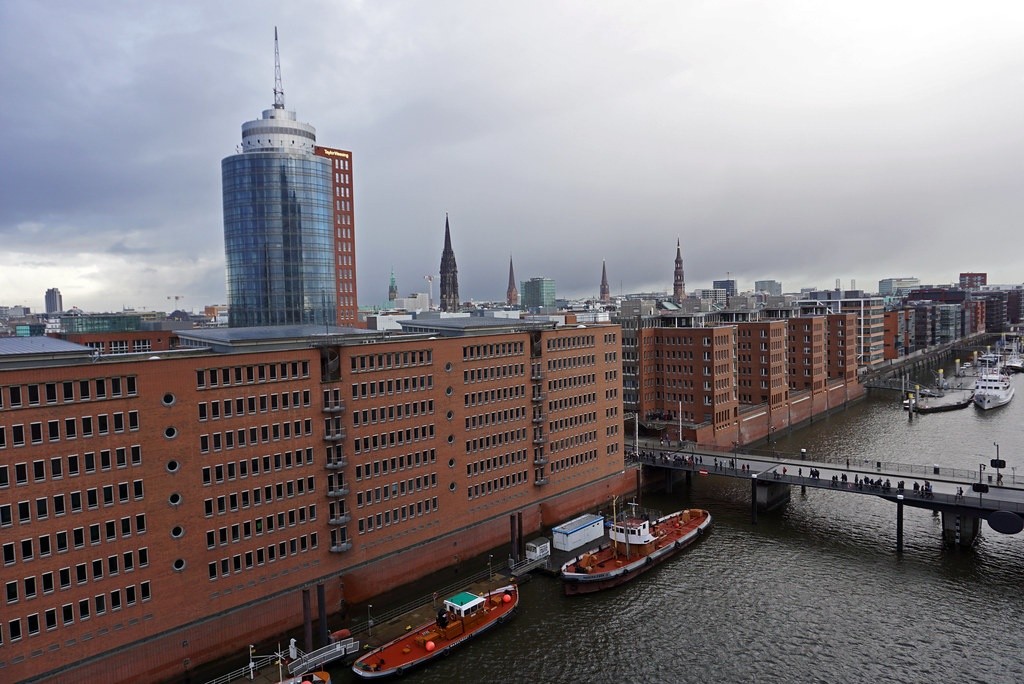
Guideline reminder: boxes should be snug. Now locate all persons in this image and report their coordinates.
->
[855,474,891,493]
[660,434,671,447]
[831,473,847,486]
[798,468,803,477]
[997,473,1003,486]
[742,464,749,473]
[645,442,647,448]
[914,481,934,499]
[575,556,584,573]
[624,449,656,464]
[773,469,777,479]
[896,481,904,494]
[646,411,672,422]
[729,459,735,469]
[660,450,671,464]
[783,467,786,476]
[673,455,703,467]
[809,468,819,480]
[713,457,722,471]
[956,487,963,498]
[436,609,449,630]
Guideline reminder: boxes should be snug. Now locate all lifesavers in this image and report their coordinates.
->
[496,617,504,624]
[443,649,450,657]
[674,541,682,549]
[698,528,704,535]
[450,613,456,621]
[647,556,653,565]
[396,666,404,676]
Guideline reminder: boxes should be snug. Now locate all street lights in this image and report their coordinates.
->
[994,441,999,474]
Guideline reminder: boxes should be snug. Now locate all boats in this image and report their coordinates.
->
[973,367,1016,409]
[977,338,1024,368]
[275,671,330,684]
[352,583,518,677]
[560,509,712,595]
